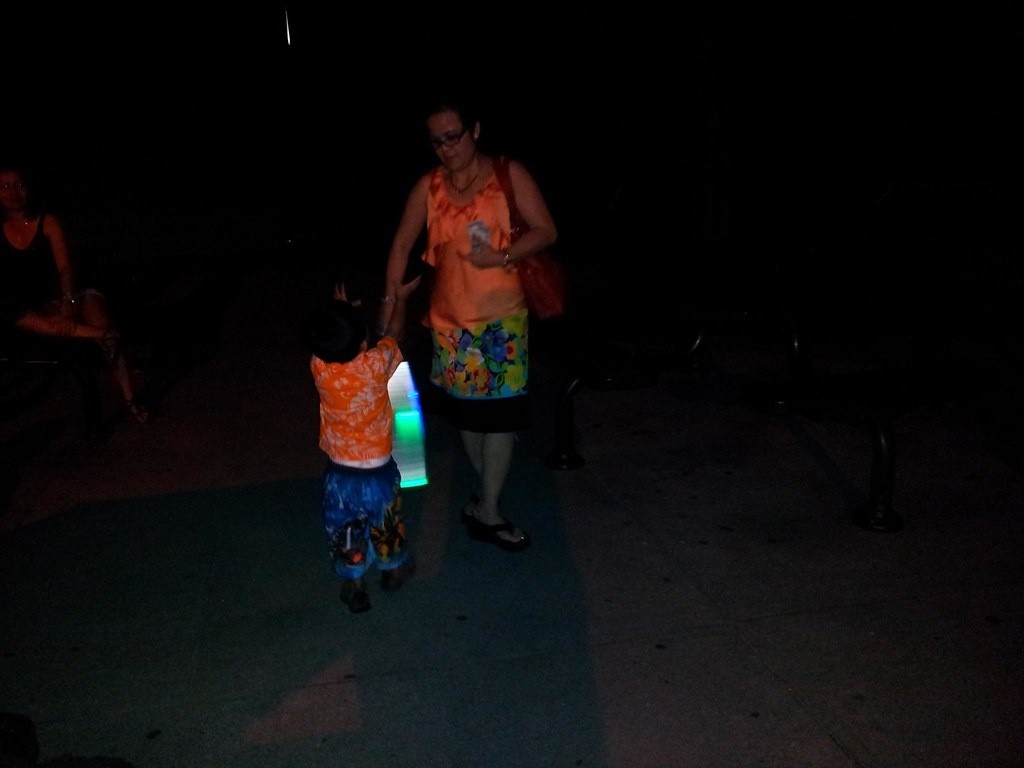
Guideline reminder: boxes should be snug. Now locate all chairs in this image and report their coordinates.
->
[0,310,110,468]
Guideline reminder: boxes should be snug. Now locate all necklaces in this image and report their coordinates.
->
[449,175,477,195]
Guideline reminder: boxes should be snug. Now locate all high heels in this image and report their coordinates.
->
[465,512,531,552]
[462,475,501,520]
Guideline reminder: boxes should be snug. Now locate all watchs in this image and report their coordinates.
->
[64,296,81,304]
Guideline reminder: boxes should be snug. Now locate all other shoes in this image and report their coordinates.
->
[381,551,416,592]
[101,326,122,362]
[117,391,156,426]
[340,577,371,614]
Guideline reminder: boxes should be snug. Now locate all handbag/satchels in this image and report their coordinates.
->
[489,155,567,317]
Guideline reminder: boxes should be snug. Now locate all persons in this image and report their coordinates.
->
[311,273,422,611]
[0,165,149,423]
[375,99,557,554]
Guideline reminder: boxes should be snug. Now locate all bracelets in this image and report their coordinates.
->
[377,326,389,336]
[382,296,396,303]
[503,248,511,266]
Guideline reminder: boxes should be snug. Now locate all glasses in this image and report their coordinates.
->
[427,124,468,150]
[0,181,28,194]
